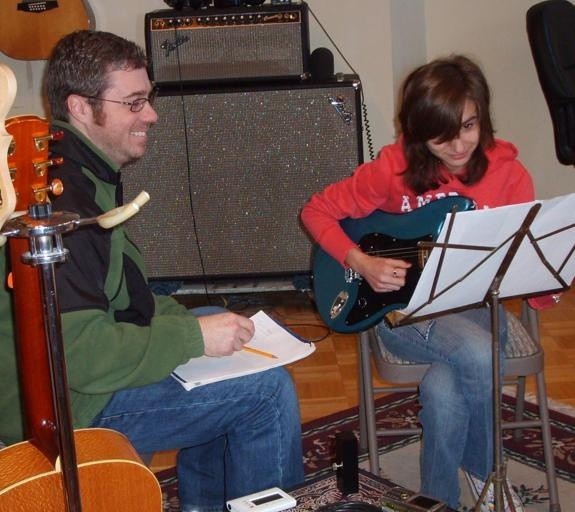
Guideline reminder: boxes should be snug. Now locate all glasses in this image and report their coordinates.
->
[77,85,156,112]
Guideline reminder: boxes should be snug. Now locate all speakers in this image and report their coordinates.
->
[144,4,311,89]
[122,73,365,283]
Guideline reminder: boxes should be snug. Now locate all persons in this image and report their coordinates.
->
[299,54,564,511]
[0,29,308,511]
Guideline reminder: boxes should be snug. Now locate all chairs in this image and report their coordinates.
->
[525,2,573,164]
[356,295,564,512]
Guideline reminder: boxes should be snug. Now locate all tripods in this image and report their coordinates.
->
[471,293,518,512]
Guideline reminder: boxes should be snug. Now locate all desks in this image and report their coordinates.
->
[229,466,465,512]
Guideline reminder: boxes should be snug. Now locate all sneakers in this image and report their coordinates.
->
[463,471,525,512]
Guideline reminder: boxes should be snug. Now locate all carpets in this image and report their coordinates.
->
[153,380,575,512]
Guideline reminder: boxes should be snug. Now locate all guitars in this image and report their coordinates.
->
[1,0,96,60]
[312,196,474,334]
[1,115,162,511]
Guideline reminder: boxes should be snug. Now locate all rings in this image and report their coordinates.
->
[393,268,397,278]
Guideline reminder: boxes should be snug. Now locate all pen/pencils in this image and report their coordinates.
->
[241,346,278,359]
